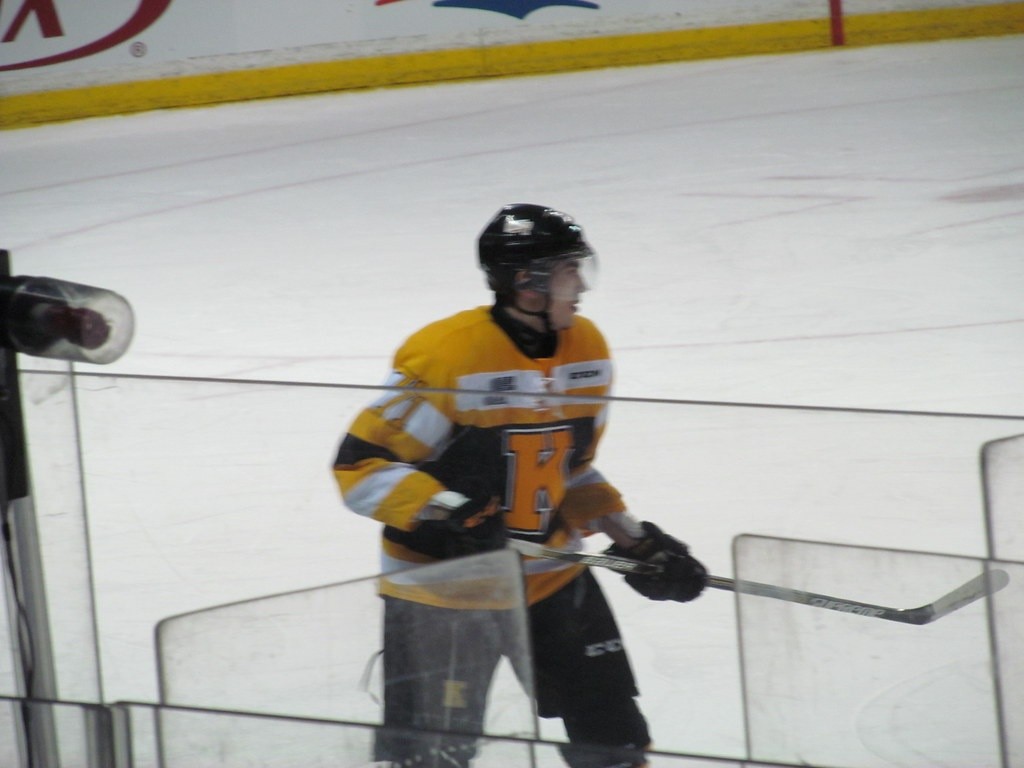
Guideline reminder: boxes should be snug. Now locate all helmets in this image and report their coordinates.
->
[478,202,592,271]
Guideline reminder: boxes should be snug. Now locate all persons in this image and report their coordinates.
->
[330,203,709,768]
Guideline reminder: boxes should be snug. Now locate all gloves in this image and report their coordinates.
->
[606,519,705,602]
[408,491,508,559]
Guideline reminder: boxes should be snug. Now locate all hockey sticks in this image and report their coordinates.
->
[453,474,1010,629]
